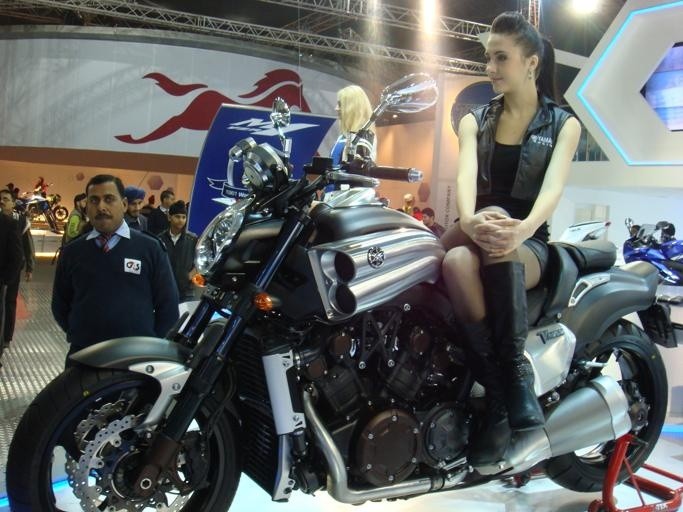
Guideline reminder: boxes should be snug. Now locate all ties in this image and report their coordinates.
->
[97,231,114,252]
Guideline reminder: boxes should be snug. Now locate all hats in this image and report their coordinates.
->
[123,185,146,203]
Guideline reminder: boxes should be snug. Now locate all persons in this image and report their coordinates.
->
[411,206,422,221]
[157,199,197,305]
[0,188,35,367]
[60,193,87,247]
[50,175,181,510]
[139,194,155,217]
[315,85,377,202]
[146,190,175,236]
[440,10,582,465]
[379,196,390,208]
[122,186,148,232]
[421,207,446,238]
[33,176,46,199]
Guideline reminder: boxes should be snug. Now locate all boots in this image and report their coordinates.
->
[463,257,546,468]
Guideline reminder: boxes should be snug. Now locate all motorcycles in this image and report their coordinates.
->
[623,217,683,287]
[14,183,69,221]
[7,72,678,512]
